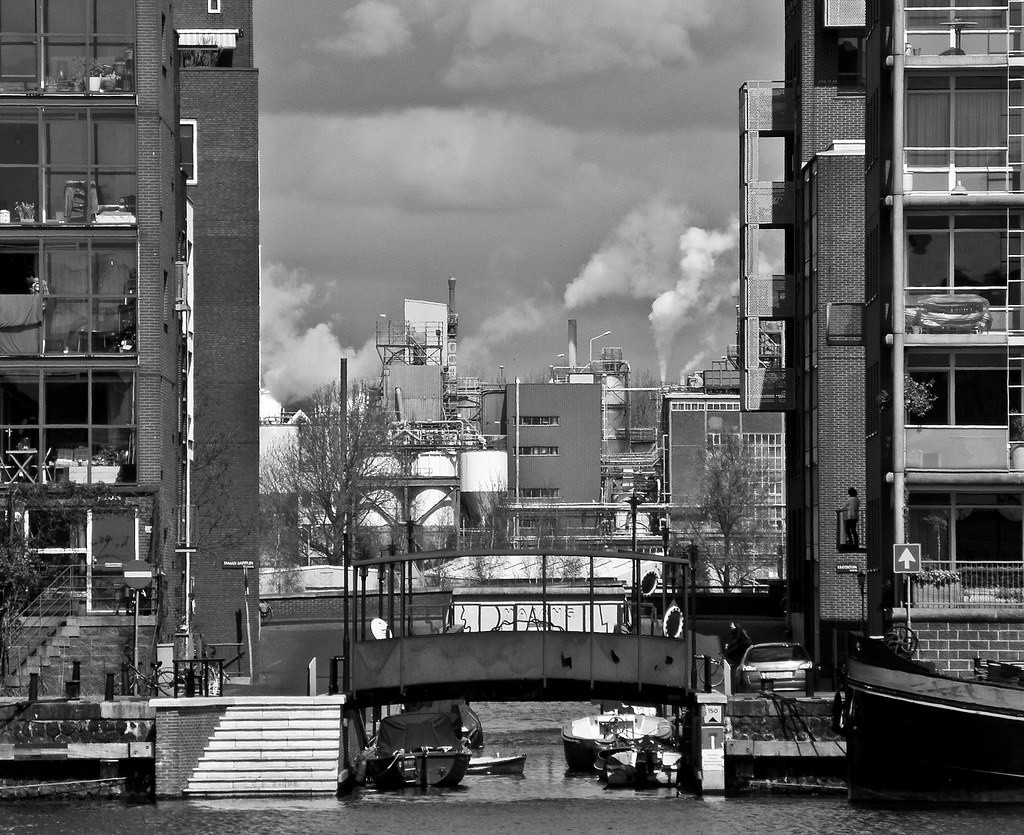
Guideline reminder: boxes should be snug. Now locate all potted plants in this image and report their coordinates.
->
[1010,409,1024,469]
[121,77,134,90]
[57,79,72,92]
[124,46,134,62]
[910,302,924,334]
[43,73,58,92]
[71,55,86,90]
[99,65,121,92]
[123,63,133,77]
[112,56,126,75]
[88,57,101,91]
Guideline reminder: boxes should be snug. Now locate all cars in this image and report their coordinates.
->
[734,642,821,697]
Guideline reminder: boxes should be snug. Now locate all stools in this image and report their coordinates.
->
[46,339,64,351]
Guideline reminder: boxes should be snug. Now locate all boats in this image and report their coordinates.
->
[657,656,725,795]
[592,715,663,787]
[724,693,847,793]
[467,753,526,775]
[560,712,672,771]
[846,625,1024,811]
[364,714,472,793]
[460,704,483,749]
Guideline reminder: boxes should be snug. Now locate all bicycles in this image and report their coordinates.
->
[261,604,273,627]
[698,651,736,688]
[113,646,187,698]
[193,659,231,696]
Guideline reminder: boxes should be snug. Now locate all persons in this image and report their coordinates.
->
[839,488,860,549]
[724,622,752,658]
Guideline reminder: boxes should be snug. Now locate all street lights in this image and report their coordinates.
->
[589,331,611,361]
[405,516,415,636]
[688,539,700,690]
[377,552,387,619]
[661,524,670,617]
[499,365,504,378]
[388,538,398,639]
[359,550,370,642]
[857,572,866,623]
[628,491,642,635]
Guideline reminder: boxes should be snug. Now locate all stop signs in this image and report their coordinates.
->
[123,559,153,589]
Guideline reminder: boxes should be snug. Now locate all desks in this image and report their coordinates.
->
[71,329,111,353]
[172,659,227,698]
[4,449,37,484]
[939,22,978,55]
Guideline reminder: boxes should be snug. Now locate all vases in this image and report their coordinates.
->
[43,588,57,599]
[912,584,965,608]
[21,219,36,223]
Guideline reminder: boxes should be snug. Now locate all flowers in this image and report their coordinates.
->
[909,567,963,584]
[12,201,34,218]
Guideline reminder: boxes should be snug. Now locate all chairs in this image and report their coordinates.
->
[31,448,53,483]
[114,195,136,214]
[0,458,14,483]
